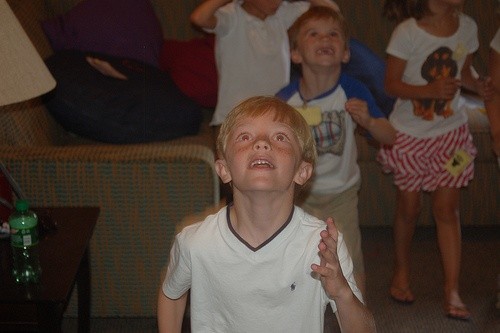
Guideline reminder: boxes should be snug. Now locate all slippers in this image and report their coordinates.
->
[388,281,415,304]
[443,297,472,320]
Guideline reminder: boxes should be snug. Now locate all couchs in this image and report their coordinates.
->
[0,0,500,316]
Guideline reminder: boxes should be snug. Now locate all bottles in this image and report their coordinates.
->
[8,200,41,290]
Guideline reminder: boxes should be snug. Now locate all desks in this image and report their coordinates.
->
[0,205,101,333]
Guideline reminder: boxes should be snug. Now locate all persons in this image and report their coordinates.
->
[187,0,500,322]
[157,97,378,332]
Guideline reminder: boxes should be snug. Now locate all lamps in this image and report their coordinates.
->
[0,0,57,110]
